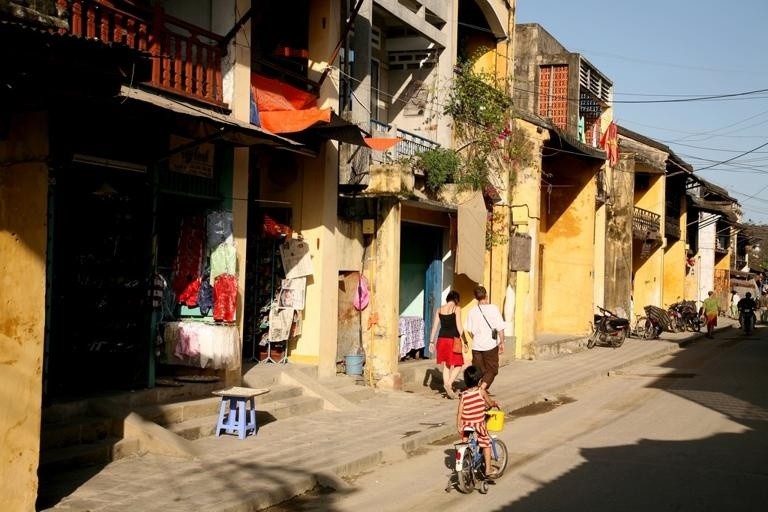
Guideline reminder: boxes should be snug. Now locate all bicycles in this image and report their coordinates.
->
[448,406,508,493]
[629,312,653,340]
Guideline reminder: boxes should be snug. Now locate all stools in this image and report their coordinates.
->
[215,396,257,440]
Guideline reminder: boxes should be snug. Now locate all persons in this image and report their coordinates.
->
[700,291,721,339]
[428,291,463,398]
[737,292,757,329]
[731,291,740,315]
[457,364,499,475]
[469,287,505,394]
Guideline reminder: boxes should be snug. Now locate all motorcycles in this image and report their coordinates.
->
[740,308,755,336]
[587,306,629,349]
[645,297,708,339]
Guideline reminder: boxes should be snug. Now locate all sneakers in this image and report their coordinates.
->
[485,466,498,476]
[443,384,460,399]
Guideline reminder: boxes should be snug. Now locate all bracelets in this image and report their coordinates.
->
[429,342,434,344]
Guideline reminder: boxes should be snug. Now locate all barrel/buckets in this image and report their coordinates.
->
[344,354,364,376]
[484,405,505,431]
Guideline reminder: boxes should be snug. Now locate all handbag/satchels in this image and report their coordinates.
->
[452,337,462,353]
[492,328,498,340]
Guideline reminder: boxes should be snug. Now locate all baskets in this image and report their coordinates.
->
[485,410,504,431]
